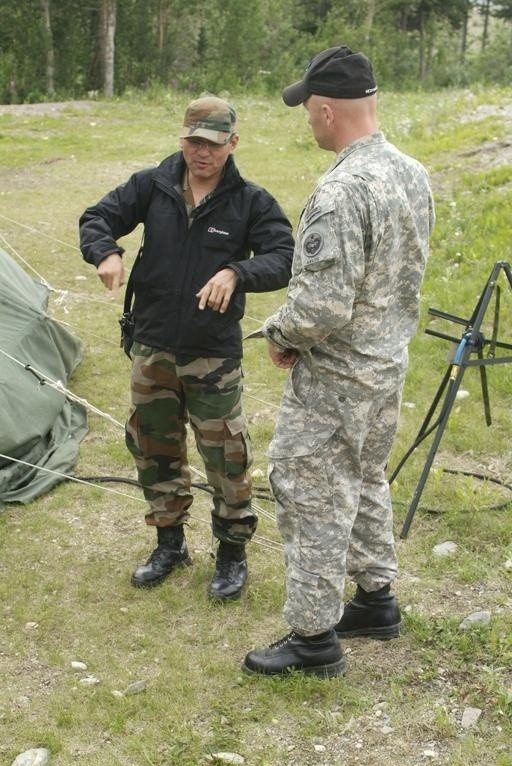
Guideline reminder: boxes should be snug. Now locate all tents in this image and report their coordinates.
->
[1,241,91,505]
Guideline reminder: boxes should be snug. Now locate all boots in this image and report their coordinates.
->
[129,525,194,590]
[205,538,250,603]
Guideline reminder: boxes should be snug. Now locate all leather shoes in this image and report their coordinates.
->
[240,628,350,683]
[333,583,403,641]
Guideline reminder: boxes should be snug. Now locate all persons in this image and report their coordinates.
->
[76,96,293,603]
[239,43,439,681]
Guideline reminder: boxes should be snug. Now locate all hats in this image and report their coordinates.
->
[281,44,378,109]
[179,96,238,146]
